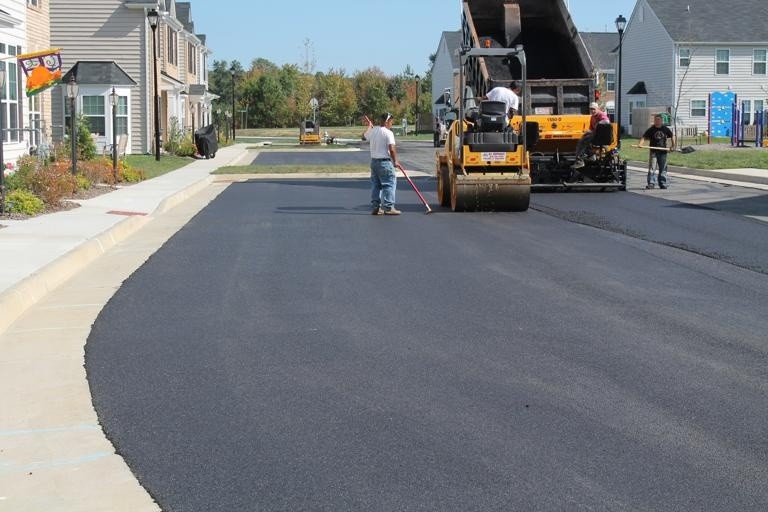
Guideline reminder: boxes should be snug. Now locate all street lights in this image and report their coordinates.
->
[611,11,629,151]
[228,63,238,142]
[106,84,119,181]
[65,70,83,179]
[411,73,421,136]
[186,99,234,154]
[145,5,163,162]
[0,56,10,221]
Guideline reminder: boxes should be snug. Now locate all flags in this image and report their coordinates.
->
[19,49,62,98]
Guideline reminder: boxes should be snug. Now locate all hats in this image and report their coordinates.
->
[588,102,599,110]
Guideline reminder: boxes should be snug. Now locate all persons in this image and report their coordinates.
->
[637,115,676,189]
[476,80,522,128]
[401,117,407,136]
[361,112,402,215]
[571,102,610,169]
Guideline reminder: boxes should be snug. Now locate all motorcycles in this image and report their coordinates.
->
[194,122,218,161]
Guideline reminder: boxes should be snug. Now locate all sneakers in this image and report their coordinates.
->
[377,206,401,215]
[660,186,667,189]
[646,185,655,189]
[569,161,585,170]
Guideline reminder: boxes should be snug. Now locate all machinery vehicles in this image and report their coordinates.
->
[430,36,538,213]
[431,87,460,149]
[297,115,322,145]
[451,0,634,195]
